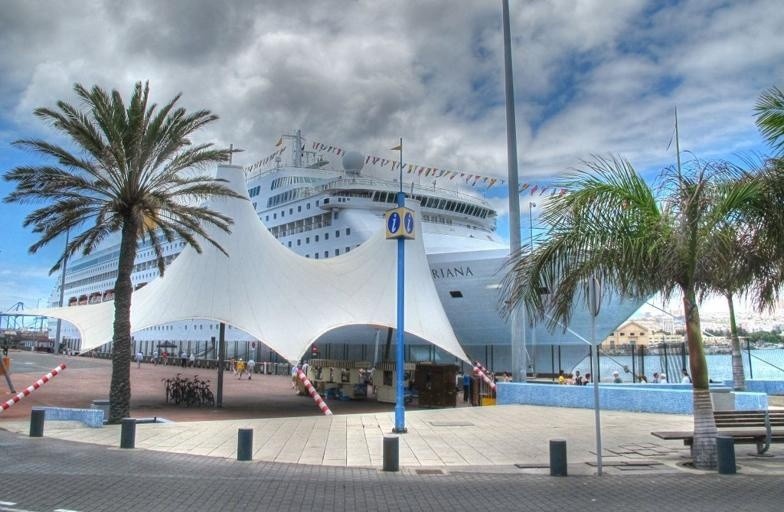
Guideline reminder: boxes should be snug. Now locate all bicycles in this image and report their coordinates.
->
[161,374,214,409]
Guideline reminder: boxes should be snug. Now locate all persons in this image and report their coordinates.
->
[455,373,463,391]
[135,350,196,369]
[302,360,310,379]
[503,371,510,382]
[558,369,591,386]
[230,356,254,380]
[639,369,692,385]
[289,366,301,388]
[461,374,471,402]
[613,370,623,383]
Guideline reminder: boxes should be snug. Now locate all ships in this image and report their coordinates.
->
[48,130,663,373]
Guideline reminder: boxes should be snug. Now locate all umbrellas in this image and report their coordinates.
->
[156,340,177,353]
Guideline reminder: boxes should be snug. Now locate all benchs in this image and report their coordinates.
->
[712,409,783,457]
